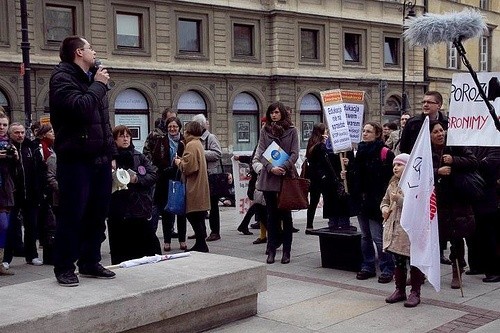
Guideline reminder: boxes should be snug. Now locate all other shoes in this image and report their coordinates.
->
[356,270,376,280]
[378,274,392,283]
[180,242,187,250]
[281,252,290,264]
[237,225,253,235]
[188,235,196,239]
[206,233,220,241]
[164,242,170,251]
[253,238,267,244]
[267,253,275,263]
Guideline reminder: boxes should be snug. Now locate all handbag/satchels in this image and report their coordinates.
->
[276,159,311,209]
[207,149,230,198]
[164,162,186,216]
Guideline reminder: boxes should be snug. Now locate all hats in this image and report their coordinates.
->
[393,153,410,166]
[192,113,207,128]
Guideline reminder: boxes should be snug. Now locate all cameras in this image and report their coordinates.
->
[2,145,15,157]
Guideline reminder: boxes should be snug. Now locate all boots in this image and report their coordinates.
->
[451,265,463,289]
[404,271,421,307]
[385,266,406,303]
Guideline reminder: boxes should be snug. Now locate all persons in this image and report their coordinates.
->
[299,90,500,308]
[174,121,210,253]
[233,101,299,265]
[0,106,59,269]
[143,108,187,251]
[188,113,221,241]
[49,37,118,286]
[107,125,158,265]
[223,172,235,207]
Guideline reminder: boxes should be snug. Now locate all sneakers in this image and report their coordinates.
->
[79,263,116,278]
[55,271,79,287]
[24,257,43,266]
[0,262,15,274]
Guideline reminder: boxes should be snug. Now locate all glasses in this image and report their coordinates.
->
[168,125,178,128]
[74,46,92,53]
[422,101,439,105]
[117,135,131,139]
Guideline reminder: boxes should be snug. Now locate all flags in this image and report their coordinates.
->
[397,117,441,293]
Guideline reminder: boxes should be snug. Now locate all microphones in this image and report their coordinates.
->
[402,10,488,47]
[95,60,111,91]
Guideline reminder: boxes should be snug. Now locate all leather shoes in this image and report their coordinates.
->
[465,270,481,274]
[440,255,452,264]
[482,275,500,282]
